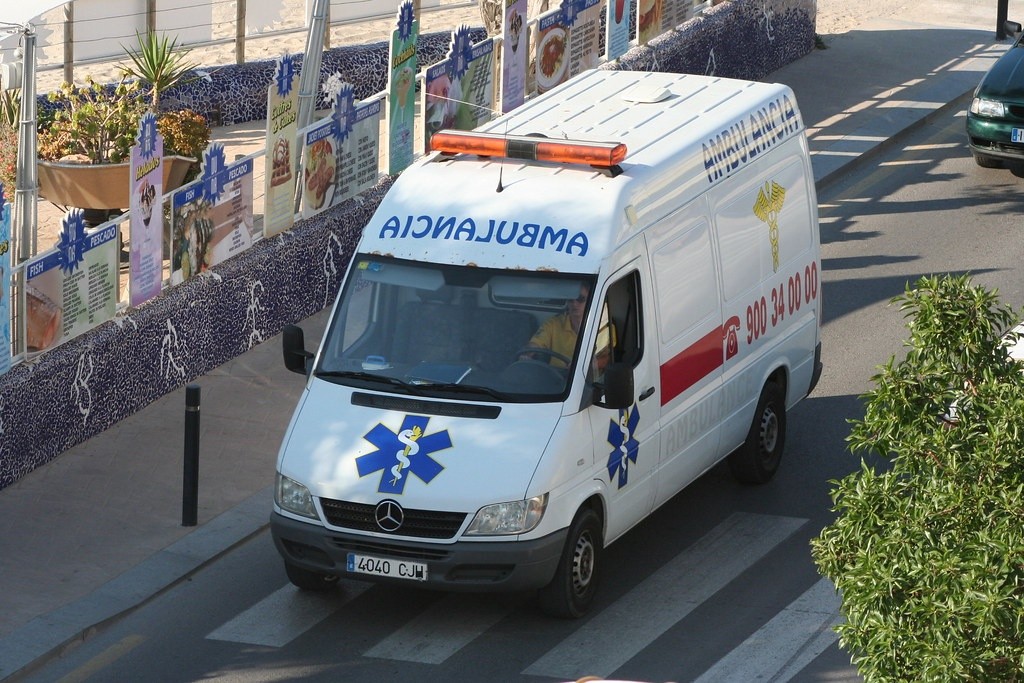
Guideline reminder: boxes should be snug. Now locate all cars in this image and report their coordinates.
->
[964,29,1024,177]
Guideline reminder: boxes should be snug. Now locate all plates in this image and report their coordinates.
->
[303,136,337,218]
[537,28,568,90]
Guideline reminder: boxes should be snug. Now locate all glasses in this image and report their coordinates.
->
[576,296,587,301]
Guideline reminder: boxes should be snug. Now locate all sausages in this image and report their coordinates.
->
[308,157,334,200]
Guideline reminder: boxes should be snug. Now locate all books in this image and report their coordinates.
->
[405,361,473,385]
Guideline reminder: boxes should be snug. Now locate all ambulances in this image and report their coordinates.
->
[271,66,825,627]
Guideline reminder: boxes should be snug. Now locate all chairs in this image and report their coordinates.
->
[390,285,467,360]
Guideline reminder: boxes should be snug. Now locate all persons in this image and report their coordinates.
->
[519,281,617,383]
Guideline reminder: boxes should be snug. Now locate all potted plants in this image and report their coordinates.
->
[35,69,198,210]
